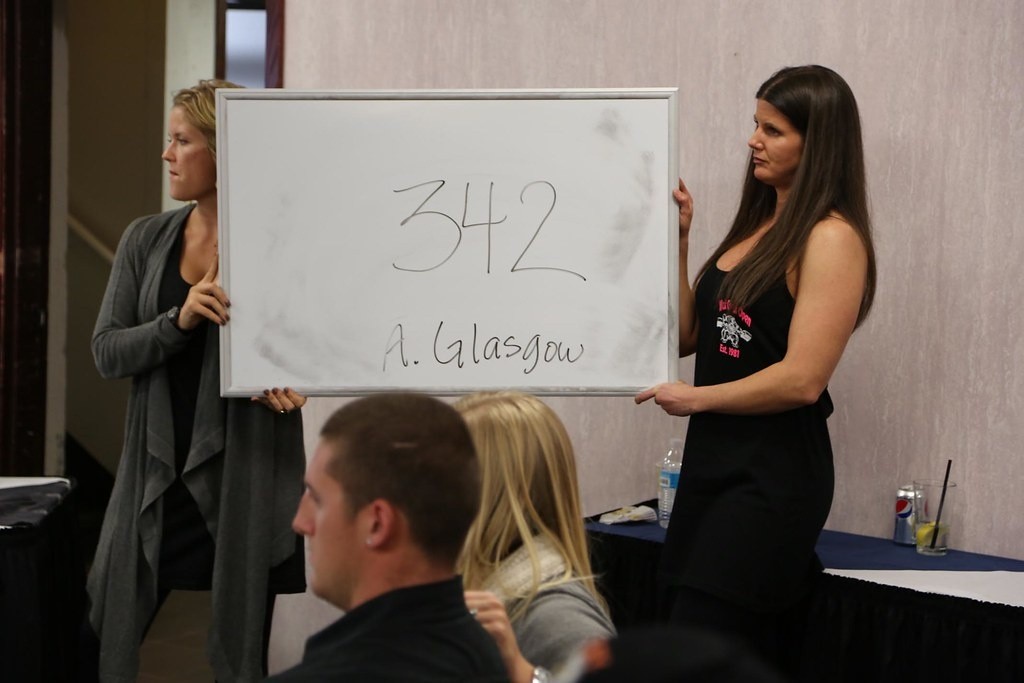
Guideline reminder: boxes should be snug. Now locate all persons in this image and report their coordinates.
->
[86,79,307,681]
[453,394,621,682]
[265,392,505,683]
[634,65,876,683]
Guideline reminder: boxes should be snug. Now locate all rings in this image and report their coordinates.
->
[467,607,478,618]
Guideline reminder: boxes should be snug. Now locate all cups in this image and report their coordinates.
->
[913,479,956,556]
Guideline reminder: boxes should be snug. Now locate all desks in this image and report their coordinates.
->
[583,496,1024,682]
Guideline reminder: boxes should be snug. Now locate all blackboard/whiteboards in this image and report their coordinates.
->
[215,87,681,398]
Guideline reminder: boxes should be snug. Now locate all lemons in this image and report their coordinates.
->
[917,522,948,545]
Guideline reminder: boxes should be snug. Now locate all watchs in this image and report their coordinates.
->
[166,305,180,325]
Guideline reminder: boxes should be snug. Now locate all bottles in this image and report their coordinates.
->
[658,439,683,528]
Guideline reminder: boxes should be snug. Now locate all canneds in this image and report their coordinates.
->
[894,485,929,546]
